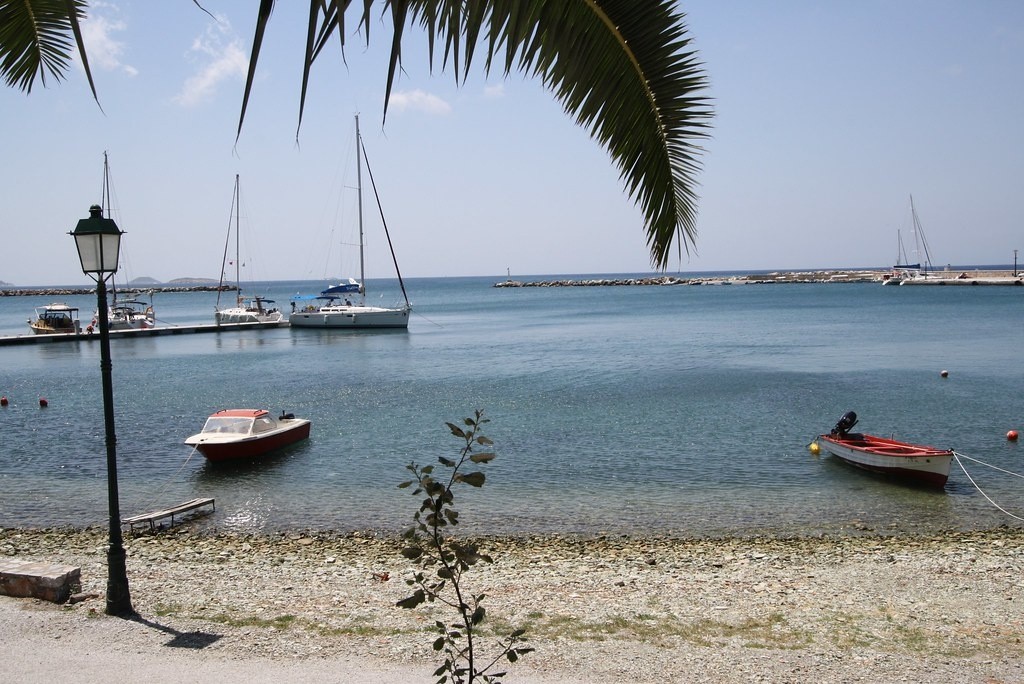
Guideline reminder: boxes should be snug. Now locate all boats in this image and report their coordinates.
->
[184,409,312,461]
[93,299,155,329]
[721,281,732,285]
[817,411,955,488]
[660,282,674,286]
[27,303,82,334]
[828,275,849,283]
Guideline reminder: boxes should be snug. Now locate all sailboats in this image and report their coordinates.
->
[288,115,412,328]
[214,174,283,321]
[909,194,941,278]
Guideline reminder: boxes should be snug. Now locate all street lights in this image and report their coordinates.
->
[67,204,132,616]
[1014,250,1018,277]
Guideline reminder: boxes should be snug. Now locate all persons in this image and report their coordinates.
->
[331,299,336,306]
[345,299,352,306]
[92,318,96,326]
[87,325,94,335]
